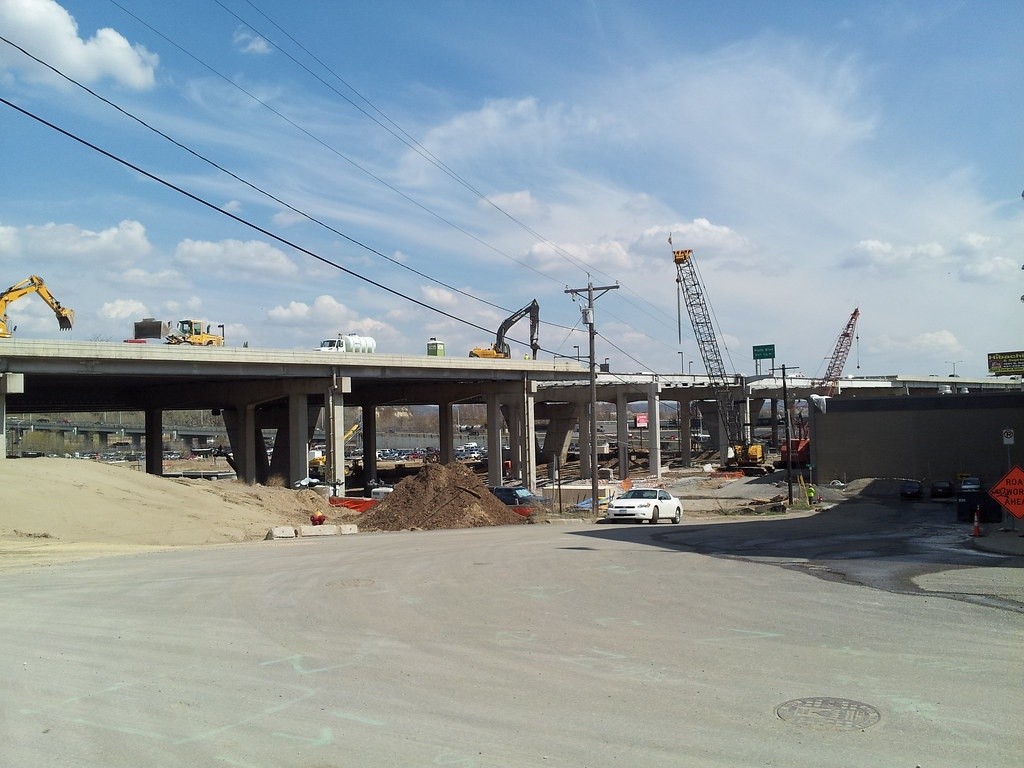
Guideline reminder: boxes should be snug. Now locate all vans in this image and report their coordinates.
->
[381,449,391,454]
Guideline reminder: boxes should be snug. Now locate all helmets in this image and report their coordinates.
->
[808,484,812,486]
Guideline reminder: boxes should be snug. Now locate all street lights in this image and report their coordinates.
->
[677,352,684,374]
[946,362,963,377]
[574,345,580,361]
[605,358,609,372]
[689,362,692,373]
[454,406,459,432]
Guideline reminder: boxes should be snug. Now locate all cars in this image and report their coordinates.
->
[933,481,956,496]
[7,416,17,419]
[606,487,685,525]
[961,477,981,493]
[36,418,50,423]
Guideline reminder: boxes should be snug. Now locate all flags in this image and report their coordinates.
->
[668,232,672,244]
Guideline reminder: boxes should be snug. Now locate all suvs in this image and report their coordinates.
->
[488,486,551,504]
[465,442,477,449]
[469,451,481,458]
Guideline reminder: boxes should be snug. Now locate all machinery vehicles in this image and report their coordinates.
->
[133,317,224,347]
[470,297,540,359]
[672,247,775,476]
[780,305,861,468]
[309,419,363,475]
[316,337,377,354]
[0,272,76,338]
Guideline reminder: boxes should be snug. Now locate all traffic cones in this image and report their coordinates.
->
[971,512,983,536]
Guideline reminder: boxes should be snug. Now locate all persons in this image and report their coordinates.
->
[524,353,530,359]
[807,482,815,505]
